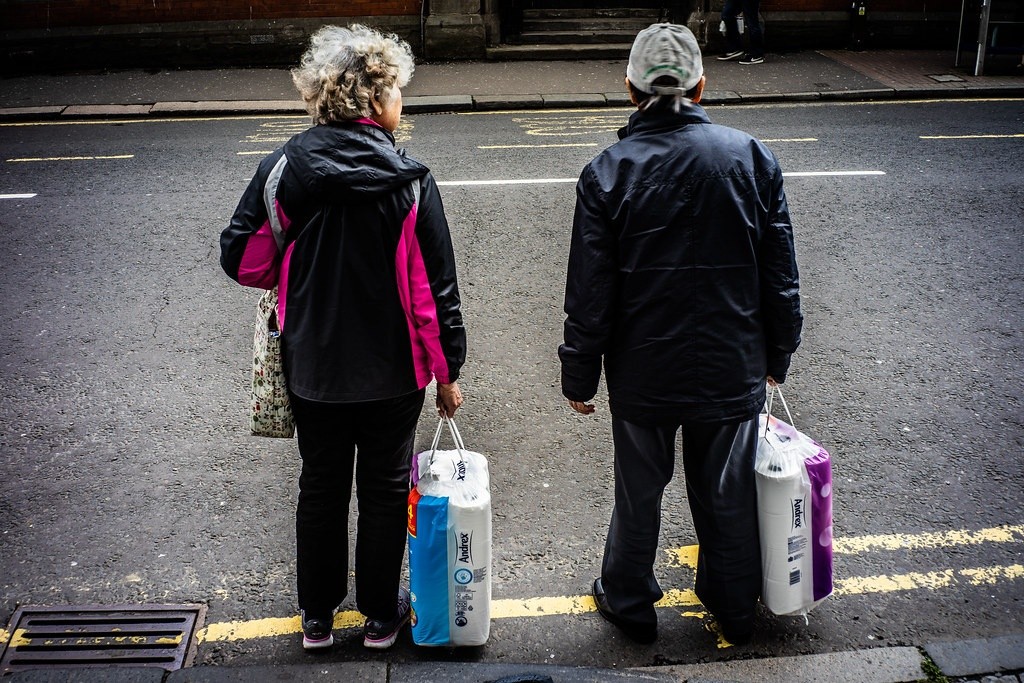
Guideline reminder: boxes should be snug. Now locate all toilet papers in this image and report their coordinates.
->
[757,411,835,618]
[406,448,494,648]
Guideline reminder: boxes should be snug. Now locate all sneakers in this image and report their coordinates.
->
[739,54,763,65]
[716,48,744,60]
[300,605,339,649]
[363,585,411,649]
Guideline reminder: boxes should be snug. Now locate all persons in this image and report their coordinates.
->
[717,0,765,64]
[560,24,802,635]
[221,27,466,646]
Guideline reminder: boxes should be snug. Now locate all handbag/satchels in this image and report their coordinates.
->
[248,284,295,438]
[407,410,492,646]
[719,16,744,34]
[754,376,834,616]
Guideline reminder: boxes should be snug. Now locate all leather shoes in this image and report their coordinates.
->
[720,621,755,647]
[592,577,658,647]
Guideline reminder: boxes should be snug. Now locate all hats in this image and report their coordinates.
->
[626,23,704,95]
